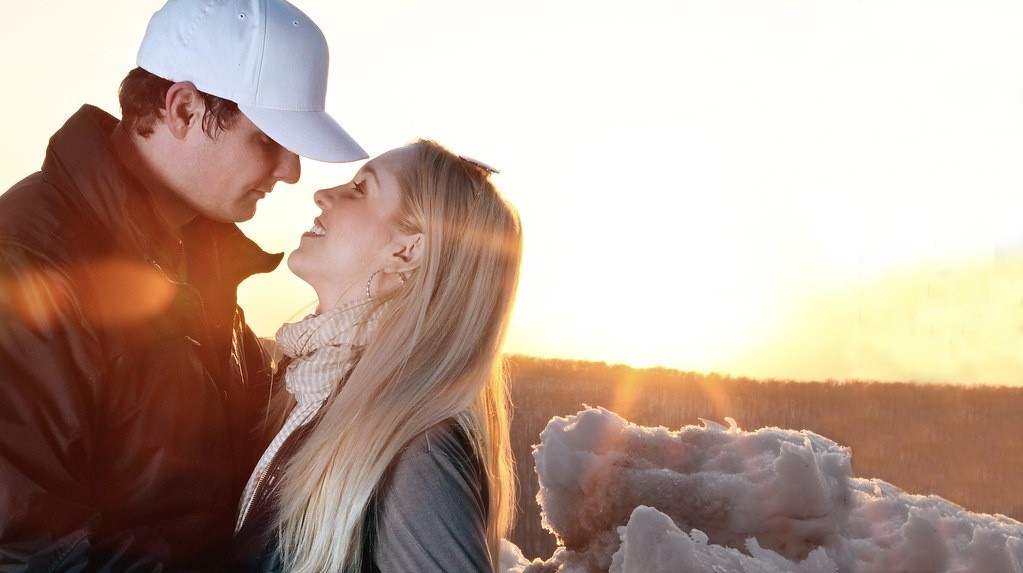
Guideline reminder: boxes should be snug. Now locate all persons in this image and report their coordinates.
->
[235,137,523,573]
[0,0,369,573]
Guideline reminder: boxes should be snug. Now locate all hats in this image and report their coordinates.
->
[137,0,371,163]
